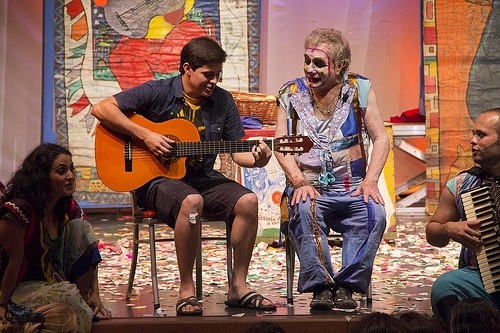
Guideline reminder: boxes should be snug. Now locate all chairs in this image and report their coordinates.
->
[285,177,372,305]
[126,191,232,317]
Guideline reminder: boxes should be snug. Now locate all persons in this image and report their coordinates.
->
[426,108,500,313]
[0,142,112,333]
[91,37,277,316]
[273,28,390,312]
[245,298,500,333]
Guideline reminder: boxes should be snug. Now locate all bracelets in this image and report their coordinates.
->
[294,180,310,189]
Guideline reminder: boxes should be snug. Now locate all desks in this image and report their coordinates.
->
[230,121,397,245]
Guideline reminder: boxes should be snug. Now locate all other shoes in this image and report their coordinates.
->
[334,288,358,308]
[310,290,333,310]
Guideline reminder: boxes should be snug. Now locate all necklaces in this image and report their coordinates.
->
[310,89,336,113]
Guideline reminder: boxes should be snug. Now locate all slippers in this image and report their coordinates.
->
[224,292,277,311]
[176,296,203,315]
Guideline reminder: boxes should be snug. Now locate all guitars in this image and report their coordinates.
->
[95,110,315,192]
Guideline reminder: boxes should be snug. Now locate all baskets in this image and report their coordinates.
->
[230,90,277,124]
